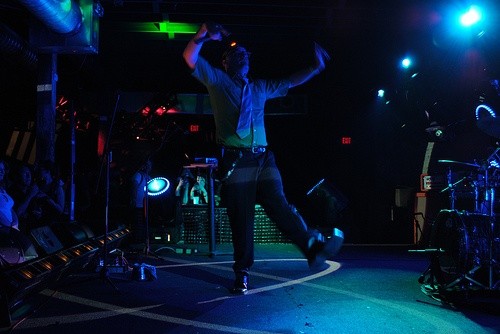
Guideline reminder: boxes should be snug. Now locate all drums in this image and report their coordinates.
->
[430,209,492,287]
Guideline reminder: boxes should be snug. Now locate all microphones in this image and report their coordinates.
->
[412,212,422,215]
[195,30,223,45]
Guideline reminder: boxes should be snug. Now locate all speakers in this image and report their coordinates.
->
[412,192,473,245]
[0,219,95,271]
[110,220,142,244]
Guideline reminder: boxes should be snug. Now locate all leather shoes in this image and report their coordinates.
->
[232,267,249,295]
[307,228,344,271]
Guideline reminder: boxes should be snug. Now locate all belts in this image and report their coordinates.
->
[223,144,268,152]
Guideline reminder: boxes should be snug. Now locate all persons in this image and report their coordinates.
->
[182,23,344,296]
[132,159,208,245]
[0,159,66,259]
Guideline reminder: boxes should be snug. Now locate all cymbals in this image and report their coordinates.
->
[438,159,481,168]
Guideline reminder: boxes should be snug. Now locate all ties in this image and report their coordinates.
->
[235,76,252,139]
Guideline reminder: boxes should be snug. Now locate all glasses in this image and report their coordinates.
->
[228,50,251,58]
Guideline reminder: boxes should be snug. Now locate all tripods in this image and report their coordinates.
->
[431,185,500,300]
